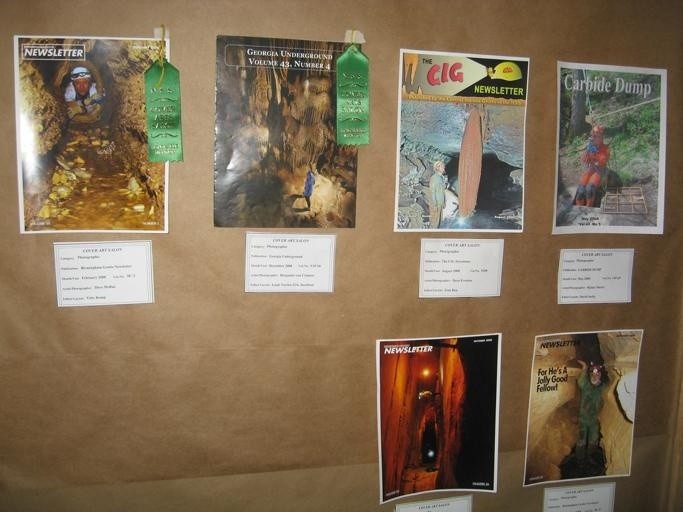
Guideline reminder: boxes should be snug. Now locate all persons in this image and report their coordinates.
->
[302,171,316,212]
[426,160,448,229]
[572,357,616,469]
[573,124,611,207]
[61,66,106,126]
[418,406,441,471]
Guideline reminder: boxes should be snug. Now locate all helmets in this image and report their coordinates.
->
[589,364,602,373]
[70,67,91,81]
